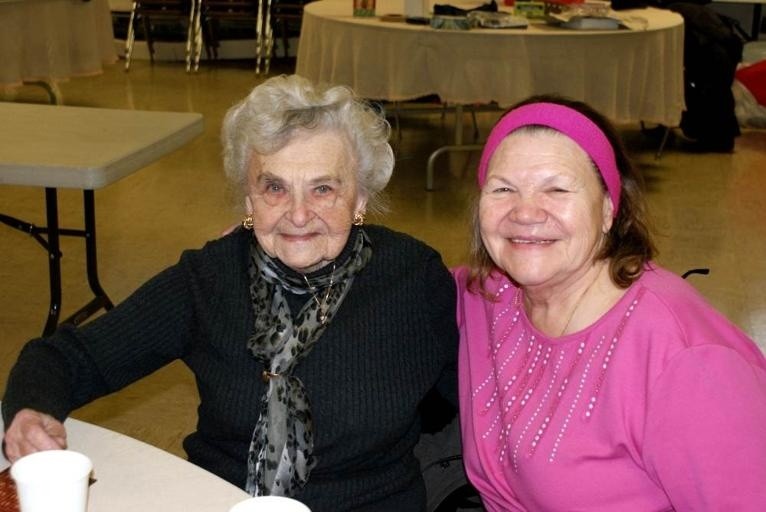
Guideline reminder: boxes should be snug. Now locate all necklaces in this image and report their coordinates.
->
[297,260,339,325]
[523,260,607,338]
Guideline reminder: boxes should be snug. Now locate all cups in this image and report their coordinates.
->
[10,450,92,512]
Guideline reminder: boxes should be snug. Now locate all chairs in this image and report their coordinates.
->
[0,77,64,105]
[123,0,315,77]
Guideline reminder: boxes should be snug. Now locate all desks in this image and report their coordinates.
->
[1,415,253,511]
[0,102,205,336]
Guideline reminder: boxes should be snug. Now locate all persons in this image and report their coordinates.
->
[0,70,463,510]
[218,88,765,511]
